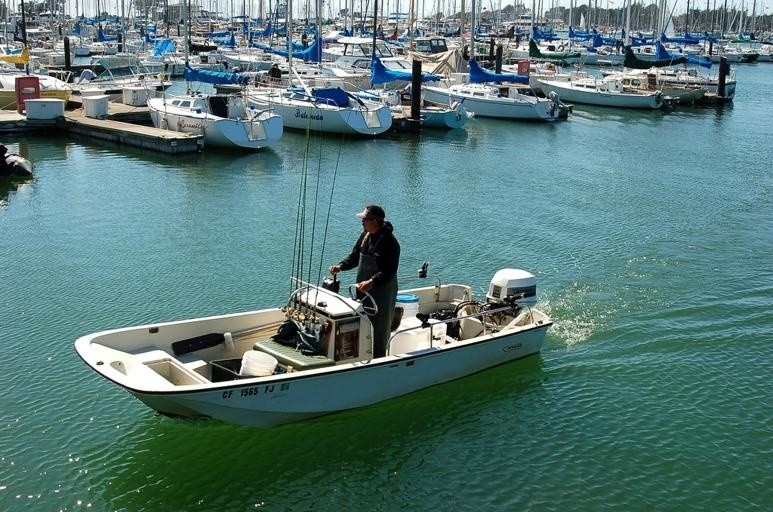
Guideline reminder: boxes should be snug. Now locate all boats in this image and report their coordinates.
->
[403,82,561,123]
[0,1,773,110]
[535,67,666,110]
[146,79,284,150]
[350,88,474,129]
[73,268,553,427]
[622,55,737,102]
[600,68,709,104]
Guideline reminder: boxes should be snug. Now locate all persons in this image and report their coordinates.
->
[267,63,282,82]
[329,203,402,357]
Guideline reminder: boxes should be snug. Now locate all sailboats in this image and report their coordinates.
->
[245,0,394,136]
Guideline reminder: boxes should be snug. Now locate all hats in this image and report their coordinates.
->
[355,206,385,219]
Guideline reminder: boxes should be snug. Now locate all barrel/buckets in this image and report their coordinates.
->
[238,348,276,377]
[395,294,420,322]
